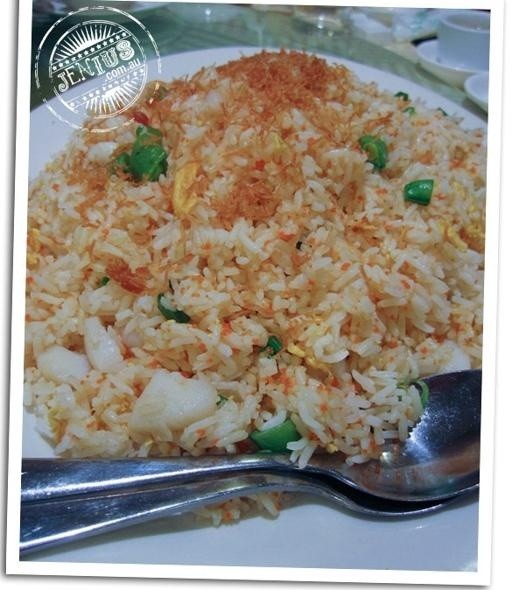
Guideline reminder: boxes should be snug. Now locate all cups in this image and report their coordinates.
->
[438,11,489,72]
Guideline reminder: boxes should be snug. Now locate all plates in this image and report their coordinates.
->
[36,1,169,25]
[464,73,488,116]
[416,39,489,91]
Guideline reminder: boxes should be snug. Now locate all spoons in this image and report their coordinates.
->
[25,366,476,561]
